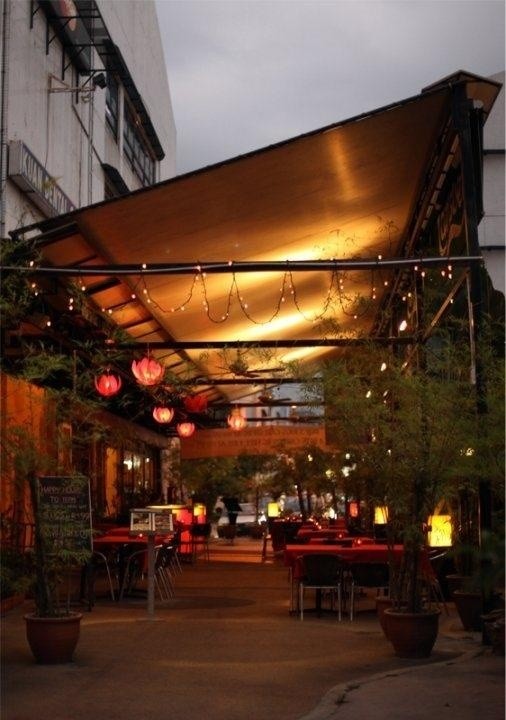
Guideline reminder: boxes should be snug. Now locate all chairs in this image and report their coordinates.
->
[262,518,406,623]
[65,520,212,613]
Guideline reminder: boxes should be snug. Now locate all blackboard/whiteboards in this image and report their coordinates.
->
[37,476,94,556]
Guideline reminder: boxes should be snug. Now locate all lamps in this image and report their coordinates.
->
[131,348,165,387]
[94,364,121,396]
[152,393,195,438]
[49,70,107,92]
[227,385,247,431]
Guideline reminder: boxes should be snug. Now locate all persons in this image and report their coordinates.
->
[345,500,376,537]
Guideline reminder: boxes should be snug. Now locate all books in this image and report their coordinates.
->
[127,510,175,536]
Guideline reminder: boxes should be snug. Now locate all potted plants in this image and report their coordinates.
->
[452,309,505,619]
[207,445,298,540]
[0,326,182,664]
[446,307,479,595]
[277,263,506,657]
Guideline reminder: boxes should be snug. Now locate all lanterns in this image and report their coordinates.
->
[129,359,166,386]
[182,394,209,414]
[175,421,195,436]
[151,407,174,424]
[226,413,248,431]
[92,374,122,396]
[348,500,358,520]
